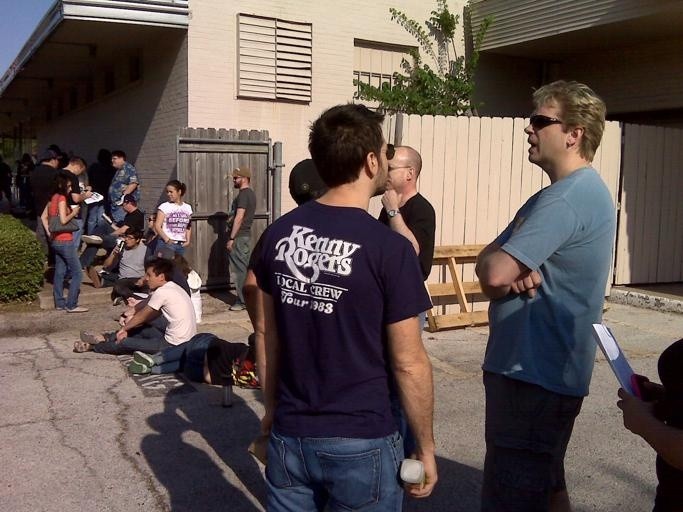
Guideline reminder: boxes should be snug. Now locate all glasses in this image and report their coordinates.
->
[529,114,560,131]
[232,177,242,179]
[124,235,134,240]
[382,144,395,159]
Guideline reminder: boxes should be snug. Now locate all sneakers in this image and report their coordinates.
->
[128,360,147,374]
[133,351,154,367]
[81,235,103,244]
[73,341,91,353]
[67,307,89,313]
[113,296,123,306]
[80,331,101,344]
[229,303,246,311]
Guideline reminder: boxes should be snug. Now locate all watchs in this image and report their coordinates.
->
[387,209,401,217]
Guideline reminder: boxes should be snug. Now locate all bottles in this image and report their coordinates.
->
[398,457,425,490]
[221,369,234,408]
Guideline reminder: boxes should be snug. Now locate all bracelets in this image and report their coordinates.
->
[229,238,234,240]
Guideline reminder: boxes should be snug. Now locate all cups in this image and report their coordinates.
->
[118,240,125,255]
[69,204,78,211]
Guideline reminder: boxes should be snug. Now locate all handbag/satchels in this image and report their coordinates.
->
[48,196,80,233]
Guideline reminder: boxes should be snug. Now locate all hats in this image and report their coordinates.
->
[289,159,323,203]
[229,167,252,178]
[40,150,63,160]
[116,194,135,205]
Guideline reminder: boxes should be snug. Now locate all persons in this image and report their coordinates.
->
[475,81,616,512]
[617,339,683,512]
[128,334,256,386]
[1,143,142,312]
[289,158,329,206]
[225,167,256,312]
[251,104,439,512]
[377,146,436,336]
[73,178,202,352]
